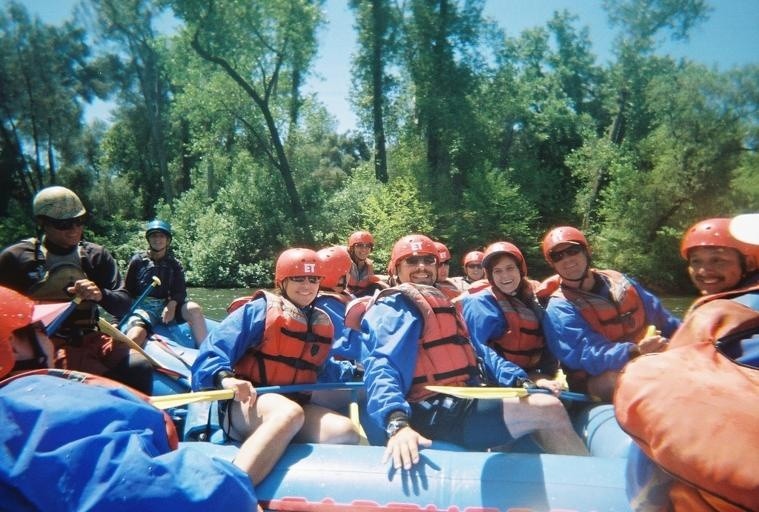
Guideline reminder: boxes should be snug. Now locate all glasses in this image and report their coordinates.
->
[549,245,581,262]
[53,215,86,230]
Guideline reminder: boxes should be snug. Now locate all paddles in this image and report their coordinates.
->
[426,386,604,401]
[150,381,363,410]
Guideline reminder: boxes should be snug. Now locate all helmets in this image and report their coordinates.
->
[543,226,592,267]
[682,219,758,272]
[387,234,450,276]
[146,220,171,238]
[274,230,373,288]
[464,242,528,278]
[33,186,86,220]
